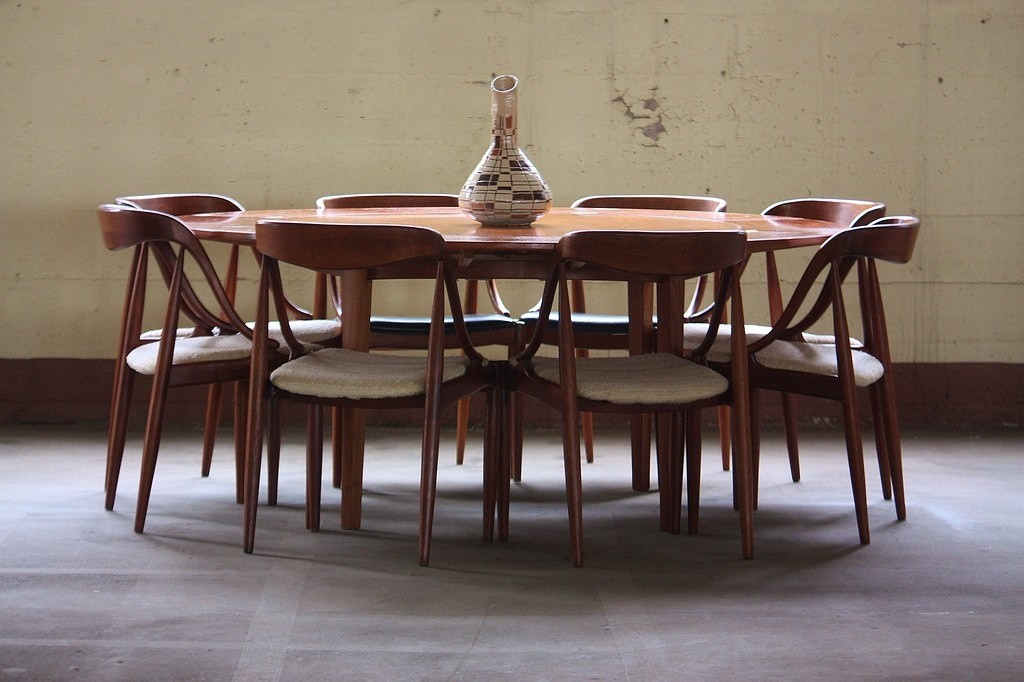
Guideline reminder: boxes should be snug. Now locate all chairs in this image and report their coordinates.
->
[97,193,921,565]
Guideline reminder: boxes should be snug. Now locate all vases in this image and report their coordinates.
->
[458,73,554,227]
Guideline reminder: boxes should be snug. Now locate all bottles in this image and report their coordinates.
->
[458,76,552,223]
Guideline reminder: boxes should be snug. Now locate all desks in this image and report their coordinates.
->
[169,207,849,537]
